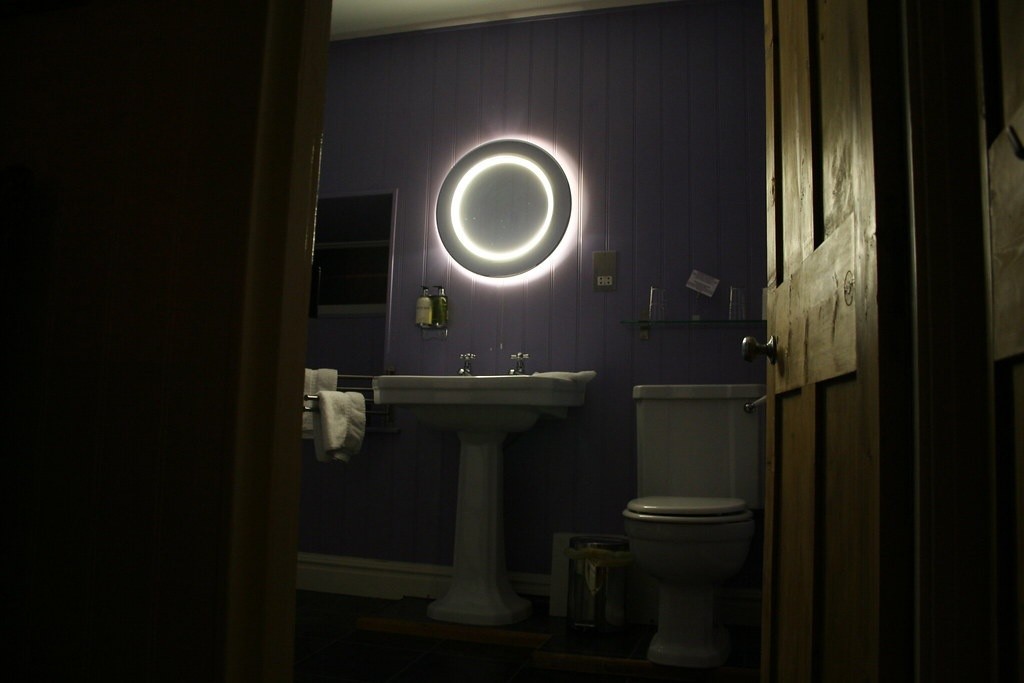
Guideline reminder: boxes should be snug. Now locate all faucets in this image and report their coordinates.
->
[457,352,478,376]
[508,353,532,375]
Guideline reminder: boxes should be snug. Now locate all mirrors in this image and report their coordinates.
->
[434,140,571,278]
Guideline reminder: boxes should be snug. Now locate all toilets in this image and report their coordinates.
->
[622,382,769,670]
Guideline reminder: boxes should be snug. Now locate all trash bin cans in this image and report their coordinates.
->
[567,535,632,628]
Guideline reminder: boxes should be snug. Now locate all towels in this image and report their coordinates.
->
[301,367,341,441]
[314,391,366,466]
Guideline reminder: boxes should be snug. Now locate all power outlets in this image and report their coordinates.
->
[591,249,617,292]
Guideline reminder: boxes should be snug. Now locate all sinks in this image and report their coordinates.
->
[371,373,592,409]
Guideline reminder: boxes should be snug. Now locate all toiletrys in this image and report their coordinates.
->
[413,282,449,330]
